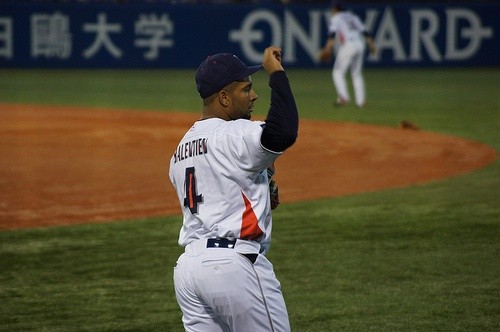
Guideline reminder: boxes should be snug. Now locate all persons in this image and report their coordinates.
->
[318,3,377,110]
[167,45,300,332]
